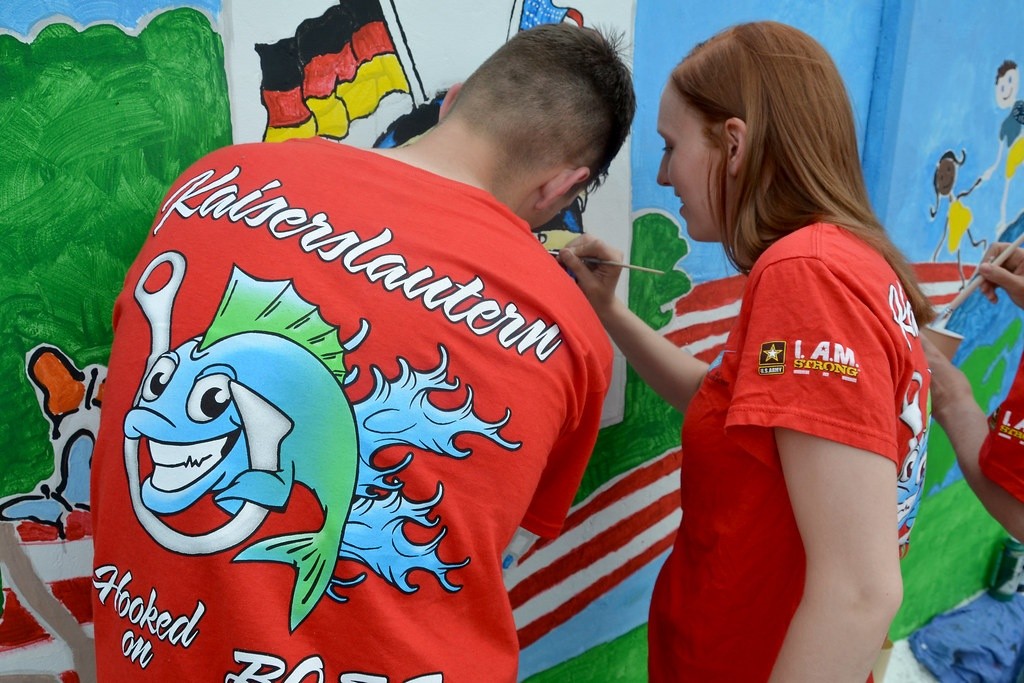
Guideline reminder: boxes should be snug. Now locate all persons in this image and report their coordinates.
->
[555,22,929,683]
[920,242,1024,546]
[89,23,635,683]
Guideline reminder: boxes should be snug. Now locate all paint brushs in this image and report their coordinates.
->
[930,231,1024,328]
[549,248,664,275]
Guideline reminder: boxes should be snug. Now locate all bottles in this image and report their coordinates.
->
[988,536,1024,601]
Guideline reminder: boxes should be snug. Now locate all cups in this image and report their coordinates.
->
[918,326,964,363]
